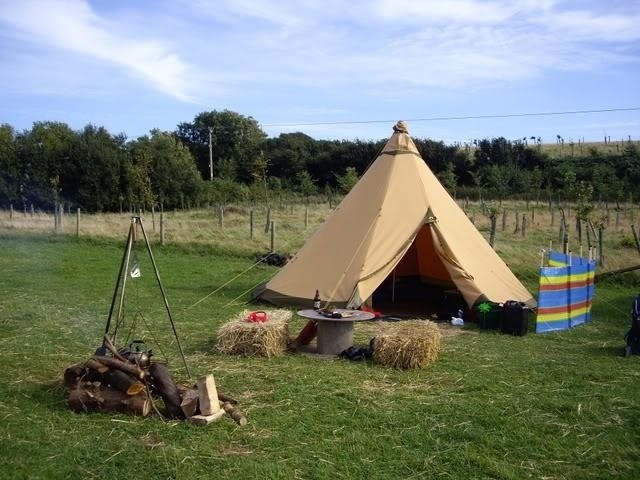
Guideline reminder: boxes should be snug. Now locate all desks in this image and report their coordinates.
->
[298,309,375,355]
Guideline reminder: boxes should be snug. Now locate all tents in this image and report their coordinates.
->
[257,120,538,313]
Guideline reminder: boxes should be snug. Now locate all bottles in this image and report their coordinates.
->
[313,289,321,310]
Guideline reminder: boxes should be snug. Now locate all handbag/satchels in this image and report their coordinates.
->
[480,310,503,330]
